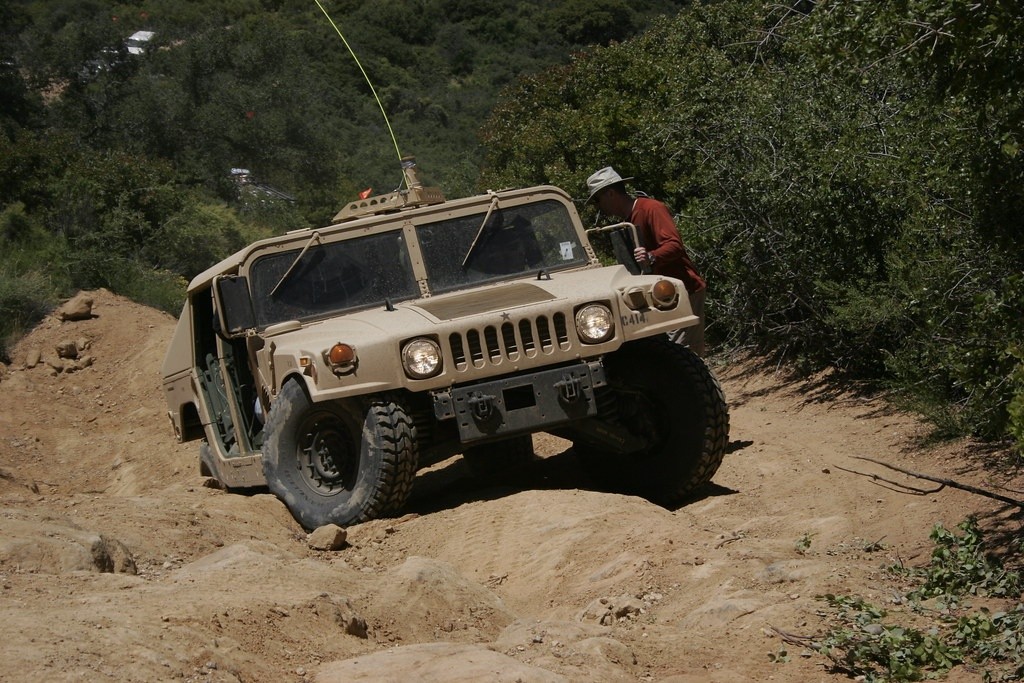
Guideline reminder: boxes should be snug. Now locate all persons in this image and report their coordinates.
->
[584,166,706,359]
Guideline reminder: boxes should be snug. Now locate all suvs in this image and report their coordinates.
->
[160,158,730,532]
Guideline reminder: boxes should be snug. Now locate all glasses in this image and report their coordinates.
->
[593,185,616,203]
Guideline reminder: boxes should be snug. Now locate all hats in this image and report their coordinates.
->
[584,166,634,206]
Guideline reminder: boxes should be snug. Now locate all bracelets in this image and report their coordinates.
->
[648,253,657,268]
